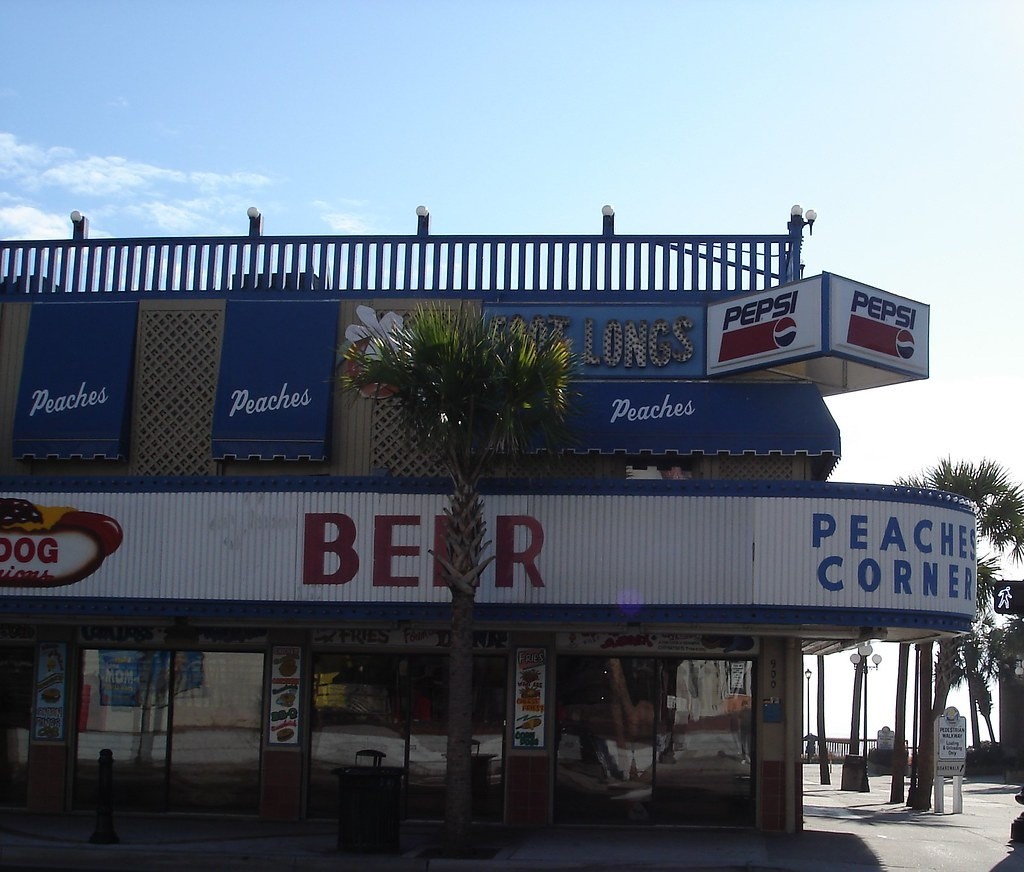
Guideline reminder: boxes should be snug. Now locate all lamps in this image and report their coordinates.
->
[247,206,260,225]
[416,206,428,224]
[70,211,83,228]
[602,204,613,223]
[792,204,817,236]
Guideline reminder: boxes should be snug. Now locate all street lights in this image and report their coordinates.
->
[850,645,882,778]
[804,668,813,759]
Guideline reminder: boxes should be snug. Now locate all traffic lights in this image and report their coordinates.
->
[994,580,1024,615]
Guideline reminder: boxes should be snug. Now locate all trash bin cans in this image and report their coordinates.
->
[334,765,406,858]
